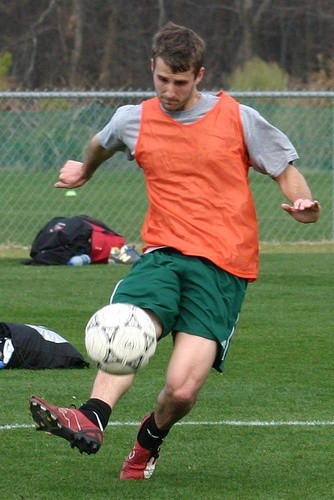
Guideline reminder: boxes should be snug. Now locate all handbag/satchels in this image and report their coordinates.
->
[0,322,89,369]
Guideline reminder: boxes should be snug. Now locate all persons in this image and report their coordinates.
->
[27,23,320,480]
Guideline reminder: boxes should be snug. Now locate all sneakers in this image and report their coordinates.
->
[30,396,101,454]
[120,413,159,479]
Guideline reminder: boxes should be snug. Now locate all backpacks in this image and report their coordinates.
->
[21,217,92,265]
[74,215,126,262]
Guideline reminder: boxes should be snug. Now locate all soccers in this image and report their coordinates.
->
[84,302,157,376]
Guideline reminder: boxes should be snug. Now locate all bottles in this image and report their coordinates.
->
[67,254,91,266]
